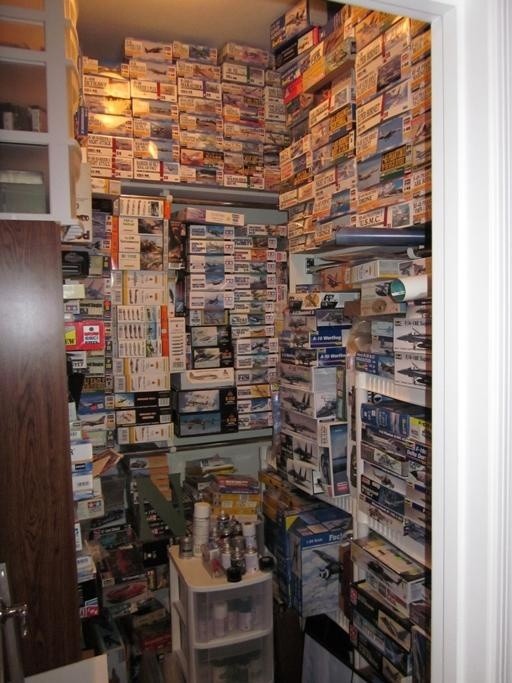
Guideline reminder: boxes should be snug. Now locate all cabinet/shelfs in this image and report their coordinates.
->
[2,0,86,218]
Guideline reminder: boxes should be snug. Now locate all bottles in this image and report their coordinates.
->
[212,598,254,637]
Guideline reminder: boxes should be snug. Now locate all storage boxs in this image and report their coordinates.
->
[69,182,433,682]
